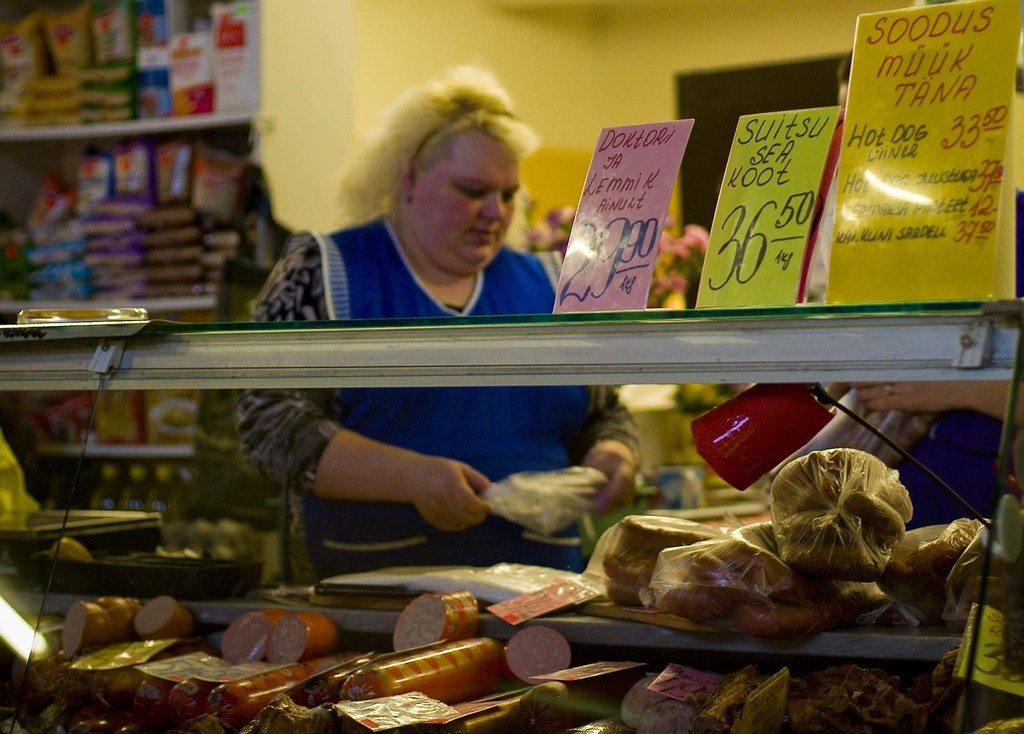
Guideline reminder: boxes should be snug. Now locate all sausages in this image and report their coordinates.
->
[0,592,634,734]
[599,447,1001,636]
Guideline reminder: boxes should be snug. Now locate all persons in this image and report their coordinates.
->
[231,69,638,574]
[769,45,1024,525]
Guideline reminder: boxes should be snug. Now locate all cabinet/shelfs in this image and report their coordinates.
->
[0,0,257,517]
